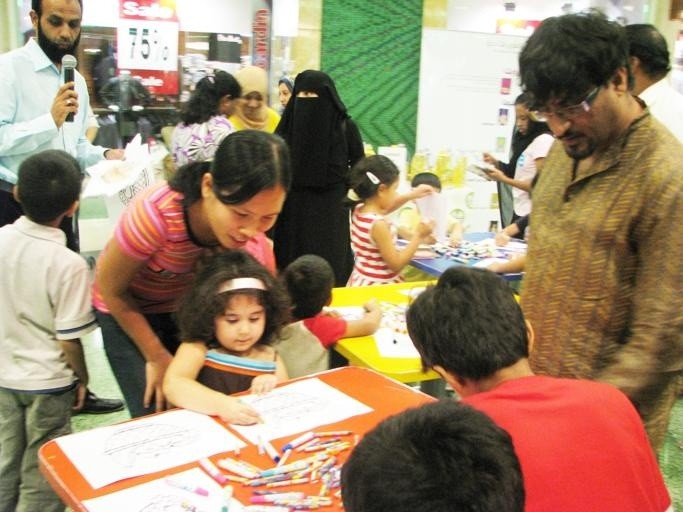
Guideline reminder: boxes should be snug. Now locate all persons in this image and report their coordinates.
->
[275,254,381,377]
[339,155,436,287]
[1,149,97,510]
[90,128,293,419]
[162,250,288,426]
[0,0,125,252]
[171,66,297,171]
[516,5,681,465]
[405,266,674,512]
[269,70,370,284]
[478,93,553,228]
[397,171,463,247]
[617,24,683,142]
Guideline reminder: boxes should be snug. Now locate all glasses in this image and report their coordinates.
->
[524,86,600,122]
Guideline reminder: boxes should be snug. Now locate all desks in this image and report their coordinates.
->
[38,365,440,511]
[328,280,521,383]
[394,230,530,279]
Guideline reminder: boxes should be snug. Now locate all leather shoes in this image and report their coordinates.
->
[72,389,124,416]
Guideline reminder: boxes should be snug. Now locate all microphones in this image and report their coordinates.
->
[62,54,77,122]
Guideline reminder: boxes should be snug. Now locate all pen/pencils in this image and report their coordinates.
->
[203,430,360,509]
[432,242,512,264]
[237,398,266,424]
[162,477,210,496]
[178,501,190,507]
